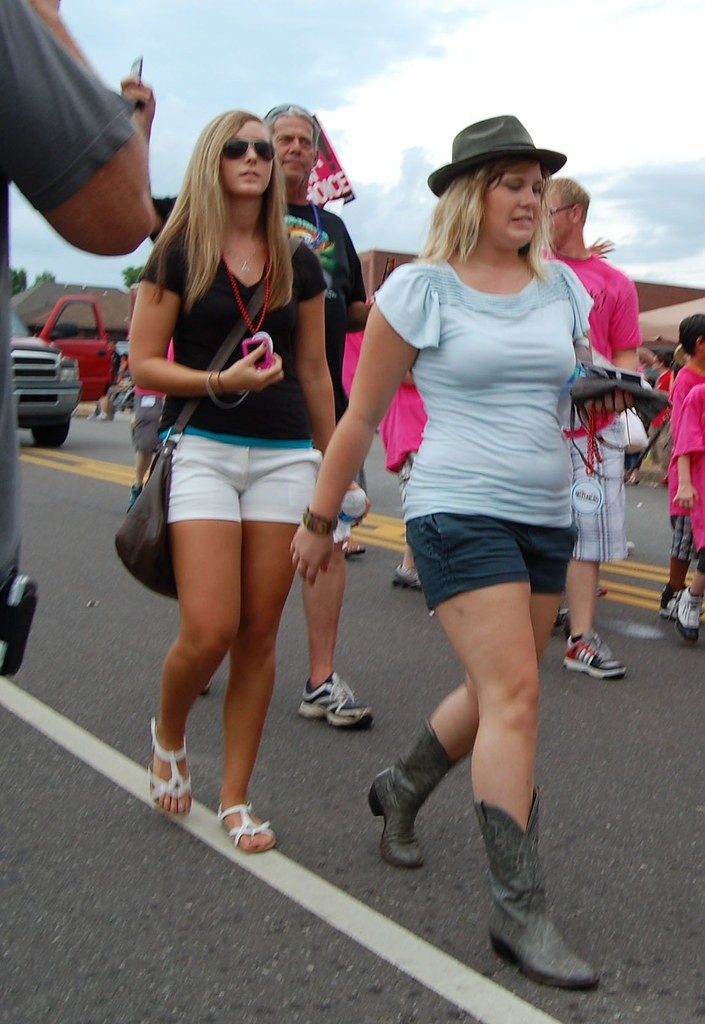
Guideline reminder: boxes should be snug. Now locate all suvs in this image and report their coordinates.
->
[11,294,118,446]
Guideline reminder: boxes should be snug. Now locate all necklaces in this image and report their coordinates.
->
[224,243,265,272]
[221,236,272,333]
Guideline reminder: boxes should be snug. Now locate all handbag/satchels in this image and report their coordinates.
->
[621,407,647,453]
[114,438,180,599]
[570,376,672,479]
[640,417,671,477]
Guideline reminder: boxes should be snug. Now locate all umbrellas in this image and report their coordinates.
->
[638,296,705,343]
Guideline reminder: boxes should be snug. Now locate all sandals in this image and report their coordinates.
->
[219,801,275,851]
[149,717,194,813]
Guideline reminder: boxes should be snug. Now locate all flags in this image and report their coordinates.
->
[303,116,356,208]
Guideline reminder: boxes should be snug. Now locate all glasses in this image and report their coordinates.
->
[223,138,277,161]
[547,203,575,217]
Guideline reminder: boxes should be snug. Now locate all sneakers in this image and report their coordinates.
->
[392,565,422,588]
[674,586,704,640]
[564,629,626,677]
[659,592,680,617]
[201,679,213,691]
[553,610,572,634]
[299,672,372,729]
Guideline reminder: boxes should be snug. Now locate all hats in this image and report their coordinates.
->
[427,116,568,197]
[650,347,674,367]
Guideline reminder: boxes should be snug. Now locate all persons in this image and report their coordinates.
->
[0,0,156,677]
[197,103,377,729]
[86,341,135,419]
[124,335,175,513]
[291,116,634,987]
[342,294,428,593]
[535,176,705,681]
[128,110,370,854]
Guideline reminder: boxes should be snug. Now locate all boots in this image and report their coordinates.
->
[471,787,600,988]
[366,719,455,868]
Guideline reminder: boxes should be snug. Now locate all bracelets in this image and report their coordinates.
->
[215,369,227,398]
[301,506,338,535]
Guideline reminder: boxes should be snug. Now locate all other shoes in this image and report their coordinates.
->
[343,538,365,557]
[596,584,607,596]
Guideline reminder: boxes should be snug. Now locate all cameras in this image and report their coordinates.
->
[243,337,273,370]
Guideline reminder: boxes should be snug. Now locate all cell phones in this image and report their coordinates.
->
[131,59,142,84]
